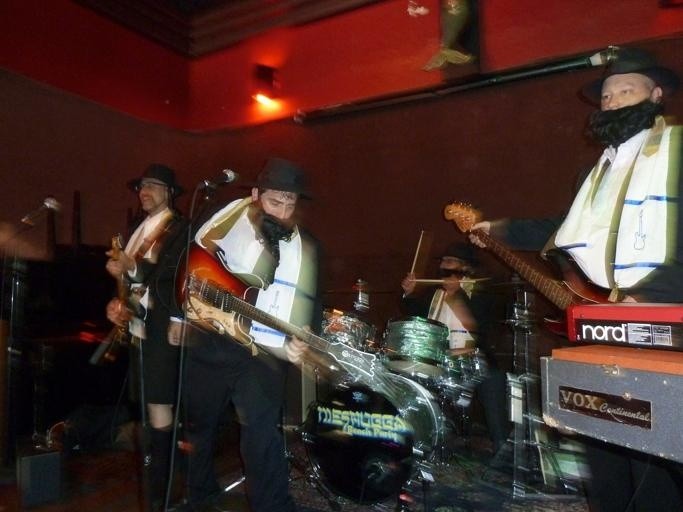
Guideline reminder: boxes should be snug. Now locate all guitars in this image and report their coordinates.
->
[173,240,376,377]
[105,234,129,360]
[444,202,617,338]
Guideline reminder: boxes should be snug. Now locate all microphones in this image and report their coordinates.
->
[19,197,57,224]
[199,168,236,192]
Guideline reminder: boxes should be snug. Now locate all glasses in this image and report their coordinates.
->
[436,267,459,276]
[134,182,167,192]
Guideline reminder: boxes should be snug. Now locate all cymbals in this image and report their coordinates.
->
[497,319,535,326]
[324,282,393,295]
[488,280,532,290]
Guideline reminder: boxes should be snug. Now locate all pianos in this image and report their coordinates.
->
[535,299,683,466]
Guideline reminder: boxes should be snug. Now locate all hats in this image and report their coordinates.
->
[579,46,674,104]
[433,240,480,265]
[239,156,312,201]
[128,163,184,199]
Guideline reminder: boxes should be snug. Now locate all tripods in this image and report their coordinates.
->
[483,289,569,500]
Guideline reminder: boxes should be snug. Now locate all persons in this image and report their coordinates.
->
[97,160,203,509]
[395,235,522,473]
[465,46,683,510]
[163,155,324,509]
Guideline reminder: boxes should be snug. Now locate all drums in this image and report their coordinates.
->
[380,313,449,369]
[302,372,440,505]
[433,348,481,396]
[314,311,380,384]
